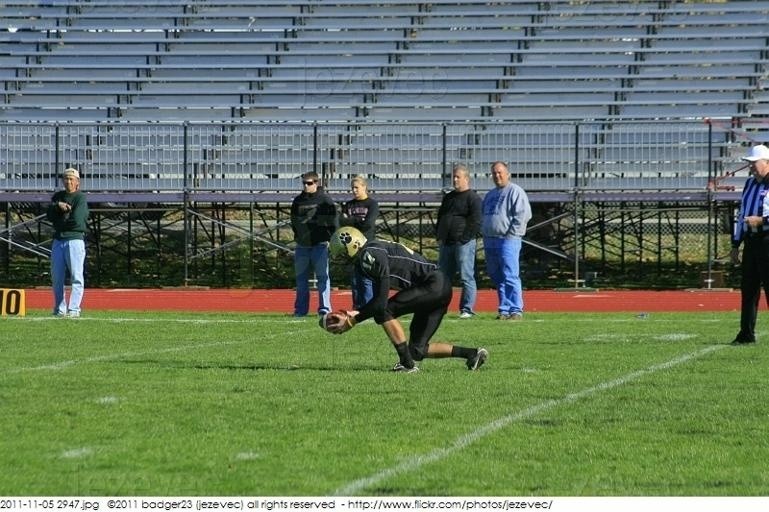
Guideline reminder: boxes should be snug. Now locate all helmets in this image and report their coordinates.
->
[327,226,368,265]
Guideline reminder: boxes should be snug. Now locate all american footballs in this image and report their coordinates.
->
[318,312,348,333]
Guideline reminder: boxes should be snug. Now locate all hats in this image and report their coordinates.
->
[740,144,769,163]
[63,168,80,179]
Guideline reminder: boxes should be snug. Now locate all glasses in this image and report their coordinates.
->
[302,181,313,186]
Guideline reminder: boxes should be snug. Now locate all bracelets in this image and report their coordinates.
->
[731,246,738,248]
[347,315,355,328]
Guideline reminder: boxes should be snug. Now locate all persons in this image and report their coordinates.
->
[435,166,484,319]
[339,177,378,317]
[327,227,488,374]
[290,171,337,317]
[480,162,532,320]
[47,168,90,317]
[731,145,768,345]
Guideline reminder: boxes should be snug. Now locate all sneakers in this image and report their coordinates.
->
[467,348,489,370]
[511,314,520,320]
[393,361,419,374]
[497,314,510,320]
[460,312,472,319]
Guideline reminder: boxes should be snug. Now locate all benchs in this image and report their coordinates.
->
[0,0,769,205]
